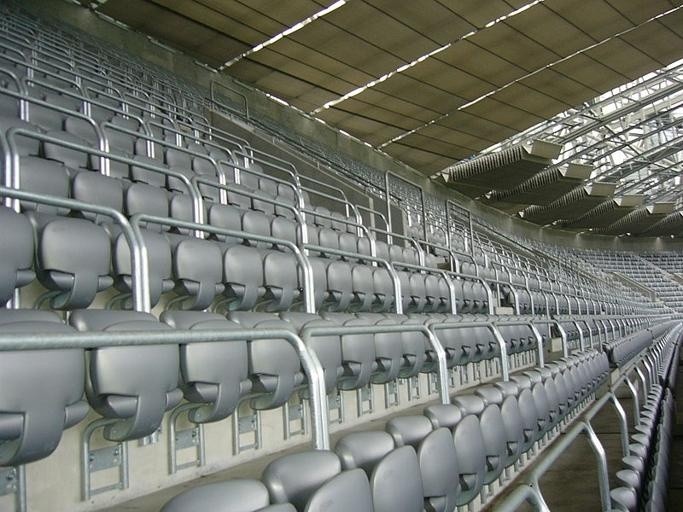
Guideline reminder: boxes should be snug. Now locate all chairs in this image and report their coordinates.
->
[1,11,683,511]
